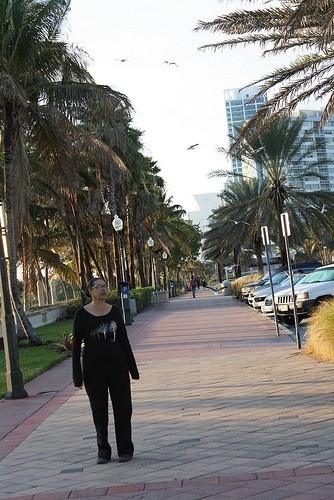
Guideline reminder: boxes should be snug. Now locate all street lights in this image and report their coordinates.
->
[147,236,157,292]
[177,263,181,290]
[111,212,126,282]
[162,251,168,291]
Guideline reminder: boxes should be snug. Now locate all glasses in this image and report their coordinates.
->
[91,284,108,289]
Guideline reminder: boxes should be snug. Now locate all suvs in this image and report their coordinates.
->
[241,262,334,325]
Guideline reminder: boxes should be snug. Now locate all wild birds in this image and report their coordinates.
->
[112,57,130,62]
[186,143,199,151]
[161,60,178,69]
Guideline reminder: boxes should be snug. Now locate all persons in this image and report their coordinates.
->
[189,275,196,298]
[197,278,200,289]
[72,277,139,465]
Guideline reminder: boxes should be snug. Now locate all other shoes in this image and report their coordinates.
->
[97,458,109,464]
[120,455,133,462]
